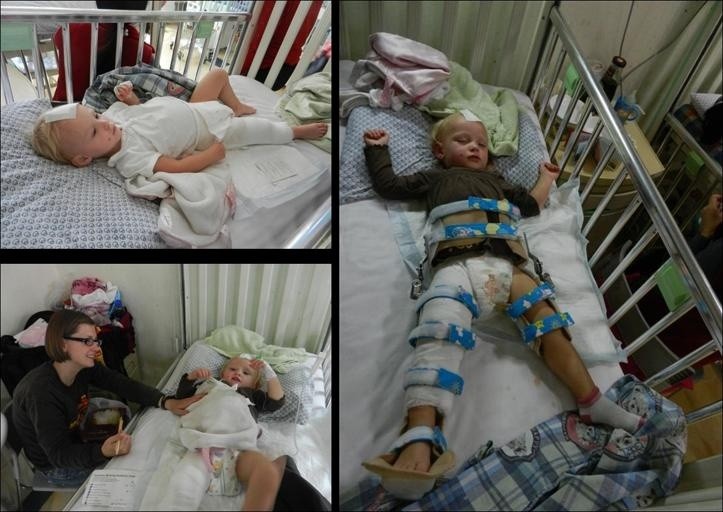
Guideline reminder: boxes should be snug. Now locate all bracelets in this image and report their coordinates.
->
[160,395,177,410]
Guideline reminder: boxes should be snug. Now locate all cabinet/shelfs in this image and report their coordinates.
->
[534,100,665,262]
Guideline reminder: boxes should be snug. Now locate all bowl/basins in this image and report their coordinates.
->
[546,94,612,145]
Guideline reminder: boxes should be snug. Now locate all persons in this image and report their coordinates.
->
[49,0,157,104]
[32,68,329,177]
[626,189,722,360]
[154,350,285,511]
[360,105,652,476]
[12,311,208,491]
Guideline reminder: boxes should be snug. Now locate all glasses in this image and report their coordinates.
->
[62,337,102,346]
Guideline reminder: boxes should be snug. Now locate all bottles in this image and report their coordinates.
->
[600,54,626,101]
[617,89,640,129]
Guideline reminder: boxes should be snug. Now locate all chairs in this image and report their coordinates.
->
[597,240,704,398]
[0,396,132,512]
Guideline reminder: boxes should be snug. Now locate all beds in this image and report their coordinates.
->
[0,1,333,251]
[62,345,331,511]
[338,2,721,511]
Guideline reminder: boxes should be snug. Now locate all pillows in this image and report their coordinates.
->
[338,102,552,208]
[164,343,315,426]
[0,99,169,248]
